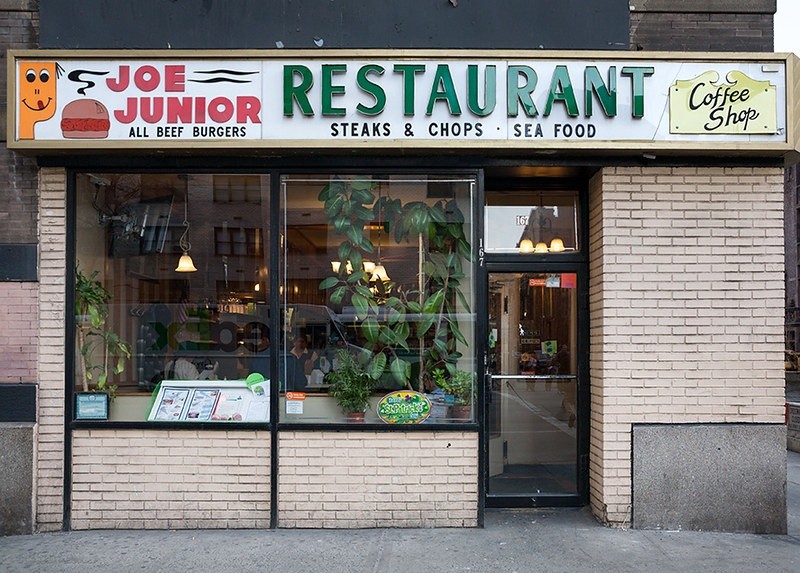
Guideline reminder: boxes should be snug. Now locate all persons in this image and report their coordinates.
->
[552,343,570,394]
[289,334,318,375]
[423,365,450,393]
[520,351,539,392]
[164,342,199,378]
[543,353,559,392]
[320,331,350,373]
[249,336,307,391]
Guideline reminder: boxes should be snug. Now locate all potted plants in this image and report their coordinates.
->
[326,349,372,423]
[432,367,478,423]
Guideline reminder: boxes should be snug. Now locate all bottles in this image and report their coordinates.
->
[197,362,204,374]
[187,298,218,350]
[229,288,243,313]
[244,299,257,315]
[218,285,228,313]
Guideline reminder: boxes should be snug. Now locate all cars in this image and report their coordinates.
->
[518,349,561,375]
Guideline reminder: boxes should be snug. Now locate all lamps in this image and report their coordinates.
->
[370,181,391,281]
[519,193,565,254]
[174,175,198,273]
[330,259,376,273]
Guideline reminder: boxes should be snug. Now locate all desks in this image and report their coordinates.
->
[305,384,335,388]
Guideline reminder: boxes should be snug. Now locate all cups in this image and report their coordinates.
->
[305,375,311,384]
[211,375,217,380]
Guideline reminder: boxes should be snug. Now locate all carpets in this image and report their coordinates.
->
[488,478,565,494]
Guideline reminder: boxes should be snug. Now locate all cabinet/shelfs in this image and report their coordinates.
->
[185,318,219,344]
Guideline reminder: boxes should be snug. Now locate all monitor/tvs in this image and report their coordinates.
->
[108,194,176,258]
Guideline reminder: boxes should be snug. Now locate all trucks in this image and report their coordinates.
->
[98,301,376,392]
